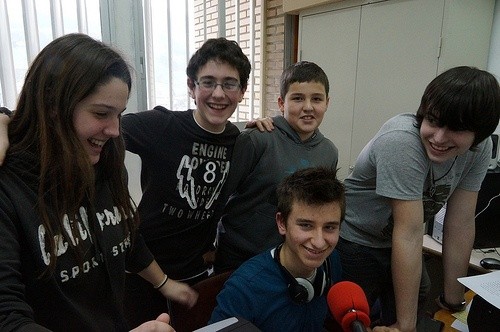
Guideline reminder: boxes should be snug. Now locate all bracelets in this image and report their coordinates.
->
[438,295,466,309]
[155,274,169,290]
[0,105,16,120]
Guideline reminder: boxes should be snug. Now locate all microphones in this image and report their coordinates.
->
[327,281,370,332]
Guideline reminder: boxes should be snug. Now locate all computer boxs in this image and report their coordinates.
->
[431,165,500,250]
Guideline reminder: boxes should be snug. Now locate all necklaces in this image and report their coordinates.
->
[430,155,459,198]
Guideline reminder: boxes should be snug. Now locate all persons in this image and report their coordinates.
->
[209,166,347,332]
[211,60,339,275]
[0,32,200,332]
[336,66,500,332]
[0,37,276,332]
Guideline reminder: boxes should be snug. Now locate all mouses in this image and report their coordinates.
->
[480,258,500,269]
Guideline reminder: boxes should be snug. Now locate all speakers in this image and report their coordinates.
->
[488,133,500,170]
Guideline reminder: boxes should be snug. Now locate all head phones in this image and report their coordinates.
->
[275,243,328,303]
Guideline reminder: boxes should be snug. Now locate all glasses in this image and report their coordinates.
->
[193,78,243,92]
